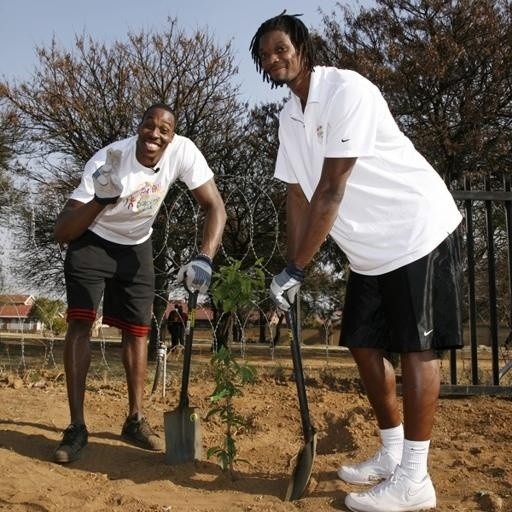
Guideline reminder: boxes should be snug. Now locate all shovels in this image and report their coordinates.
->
[282,290,317,500]
[163,273,201,461]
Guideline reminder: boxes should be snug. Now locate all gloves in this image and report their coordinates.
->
[177,254,213,294]
[269,265,305,312]
[92,148,124,205]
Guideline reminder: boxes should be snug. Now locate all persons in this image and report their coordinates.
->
[167,304,188,345]
[53,103,229,463]
[248,10,464,512]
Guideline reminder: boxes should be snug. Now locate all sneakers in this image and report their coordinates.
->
[120,416,162,451]
[54,425,89,462]
[337,444,437,512]
